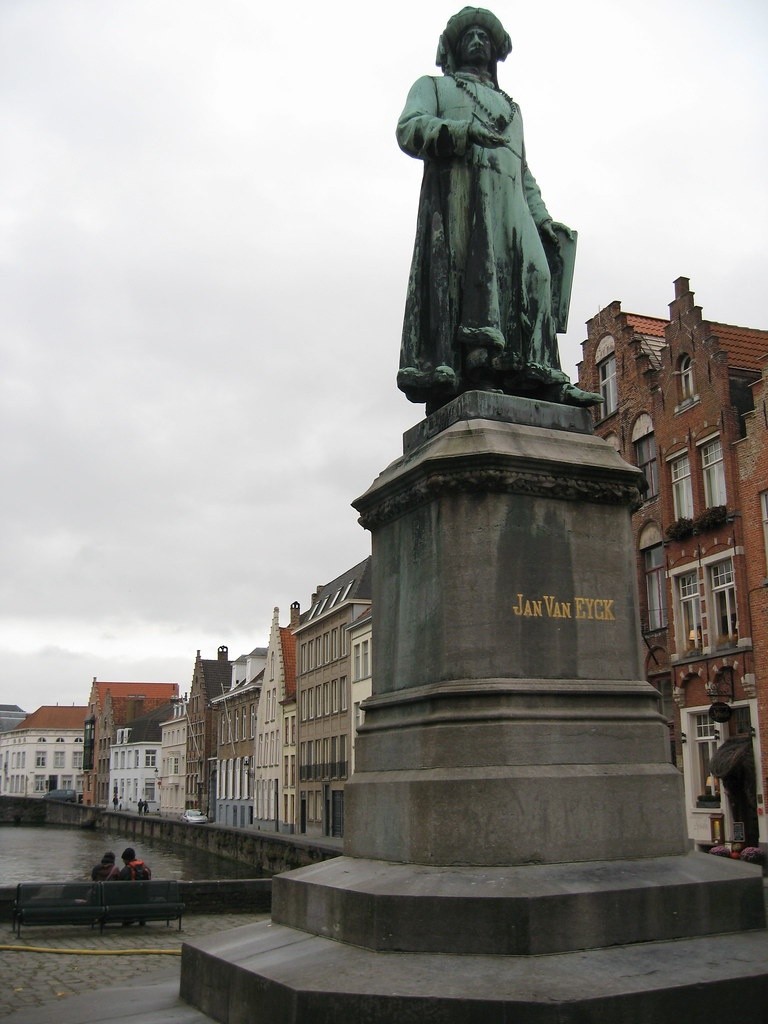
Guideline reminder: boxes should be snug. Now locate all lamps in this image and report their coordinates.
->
[154,768,160,779]
[244,760,250,774]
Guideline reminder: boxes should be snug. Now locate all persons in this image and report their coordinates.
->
[143,800,148,816]
[91,848,151,928]
[112,796,123,811]
[397,5,605,415]
[138,799,144,816]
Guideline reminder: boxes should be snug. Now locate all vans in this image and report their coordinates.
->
[40,789,77,803]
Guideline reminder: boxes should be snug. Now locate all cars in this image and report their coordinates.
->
[180,808,209,824]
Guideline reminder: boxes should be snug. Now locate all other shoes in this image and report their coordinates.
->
[139,920,145,926]
[122,920,134,926]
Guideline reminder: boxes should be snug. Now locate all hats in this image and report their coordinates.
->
[122,848,135,860]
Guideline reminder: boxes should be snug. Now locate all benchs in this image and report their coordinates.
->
[13,879,185,940]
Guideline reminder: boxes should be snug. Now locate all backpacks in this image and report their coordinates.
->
[126,865,151,881]
